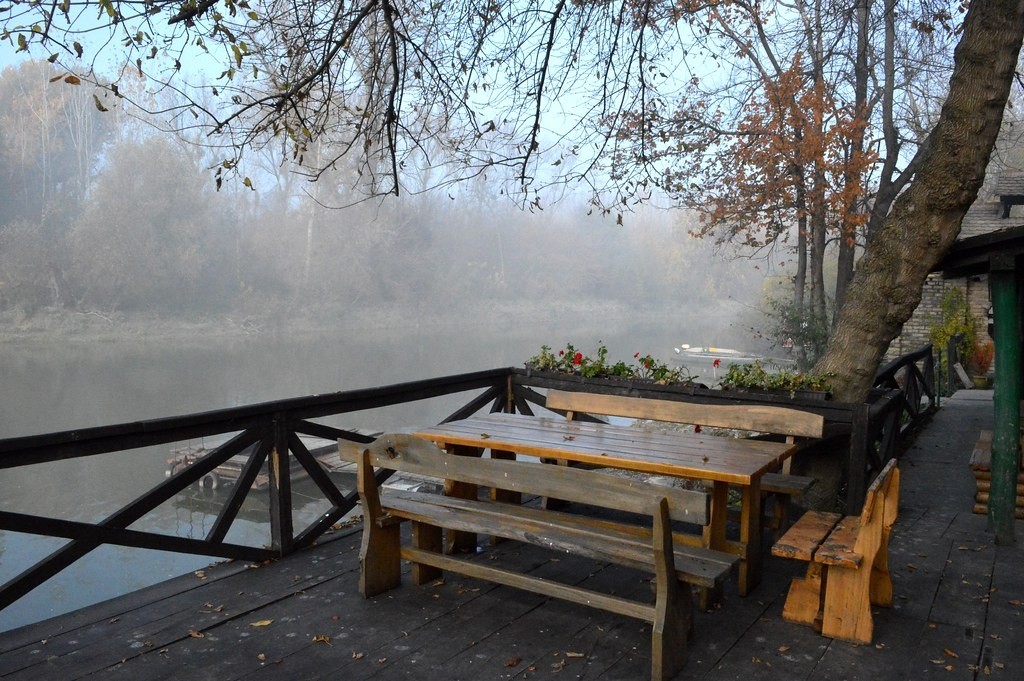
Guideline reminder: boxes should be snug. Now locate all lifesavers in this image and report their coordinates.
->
[198,471,221,490]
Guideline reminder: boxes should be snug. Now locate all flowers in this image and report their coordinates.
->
[522,342,828,391]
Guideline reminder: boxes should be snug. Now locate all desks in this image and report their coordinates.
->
[413,412,798,607]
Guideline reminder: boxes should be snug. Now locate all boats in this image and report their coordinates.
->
[674,344,764,365]
[168,416,382,491]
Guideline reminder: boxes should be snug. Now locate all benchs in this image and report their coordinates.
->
[771,459,900,646]
[335,433,736,680]
[542,388,825,596]
[969,429,1024,518]
